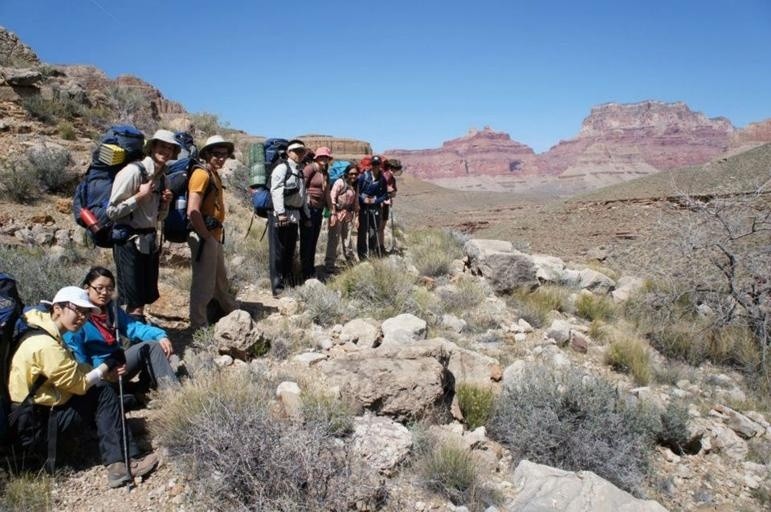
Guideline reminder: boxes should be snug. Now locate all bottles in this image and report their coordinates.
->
[175,195,187,212]
[78,204,106,235]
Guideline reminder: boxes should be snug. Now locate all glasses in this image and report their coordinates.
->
[87,283,114,294]
[210,151,228,157]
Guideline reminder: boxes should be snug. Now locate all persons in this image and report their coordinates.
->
[61,266,182,396]
[6,286,159,489]
[267,139,402,295]
[184,134,235,333]
[106,129,181,319]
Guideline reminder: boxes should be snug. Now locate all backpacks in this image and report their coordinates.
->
[0,272,48,446]
[253,138,290,217]
[161,131,212,242]
[73,126,164,248]
[323,160,351,217]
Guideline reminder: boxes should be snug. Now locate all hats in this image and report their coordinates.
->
[144,129,181,160]
[41,286,102,316]
[288,142,305,151]
[199,135,234,159]
[370,156,381,164]
[313,147,332,160]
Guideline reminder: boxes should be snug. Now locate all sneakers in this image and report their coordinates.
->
[108,453,158,488]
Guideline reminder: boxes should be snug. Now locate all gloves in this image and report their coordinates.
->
[104,349,126,371]
[274,214,290,228]
